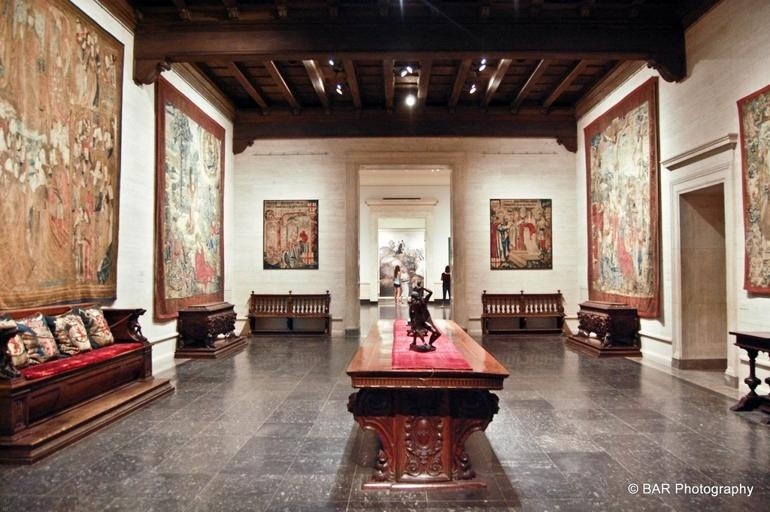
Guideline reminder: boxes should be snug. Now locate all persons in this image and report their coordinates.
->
[441,266,450,300]
[393,265,401,302]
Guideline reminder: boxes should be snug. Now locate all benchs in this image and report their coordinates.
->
[244,290,332,336]
[480,290,566,336]
[0,309,176,465]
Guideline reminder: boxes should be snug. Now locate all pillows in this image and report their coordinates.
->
[75,303,116,349]
[15,314,60,363]
[6,334,29,367]
[45,307,93,356]
